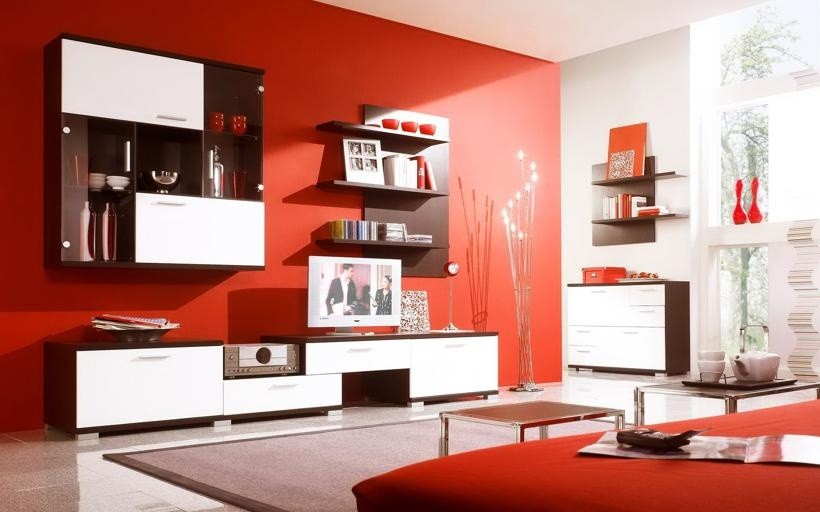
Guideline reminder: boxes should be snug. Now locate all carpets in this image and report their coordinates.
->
[101,409,642,512]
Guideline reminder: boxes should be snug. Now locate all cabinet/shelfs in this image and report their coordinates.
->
[40,330,503,435]
[39,31,269,274]
[312,116,450,257]
[565,278,690,377]
[589,171,690,226]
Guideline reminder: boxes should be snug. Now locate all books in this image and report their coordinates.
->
[383,154,437,190]
[602,193,666,219]
[328,219,433,244]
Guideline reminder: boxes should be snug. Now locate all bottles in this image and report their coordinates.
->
[731,178,747,225]
[205,144,225,198]
[747,176,766,222]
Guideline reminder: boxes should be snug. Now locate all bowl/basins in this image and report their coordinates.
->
[146,168,182,194]
[420,123,437,135]
[89,172,106,191]
[106,175,131,190]
[96,325,176,342]
[401,120,419,132]
[381,118,400,130]
[208,110,226,133]
[698,350,725,383]
[228,113,249,136]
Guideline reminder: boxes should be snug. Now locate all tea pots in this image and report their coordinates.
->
[728,325,780,383]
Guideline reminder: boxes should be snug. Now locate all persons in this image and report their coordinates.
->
[374,274,392,315]
[326,264,358,315]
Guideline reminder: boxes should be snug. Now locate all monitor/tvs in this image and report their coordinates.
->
[307,256,402,336]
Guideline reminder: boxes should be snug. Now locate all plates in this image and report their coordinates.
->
[614,277,670,283]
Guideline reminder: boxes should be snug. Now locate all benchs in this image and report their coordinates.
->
[349,397,819,512]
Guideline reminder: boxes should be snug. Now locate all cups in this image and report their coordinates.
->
[230,169,248,199]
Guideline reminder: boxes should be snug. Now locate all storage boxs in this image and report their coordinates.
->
[579,265,629,285]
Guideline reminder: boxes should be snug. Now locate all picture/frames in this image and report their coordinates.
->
[341,134,386,186]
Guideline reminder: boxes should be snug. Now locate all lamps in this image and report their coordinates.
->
[443,260,460,333]
[498,147,550,391]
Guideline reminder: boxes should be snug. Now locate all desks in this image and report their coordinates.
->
[433,397,628,456]
[630,375,820,426]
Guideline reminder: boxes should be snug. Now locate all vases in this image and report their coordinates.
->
[101,201,120,263]
[78,199,97,261]
[734,179,747,224]
[747,176,764,225]
